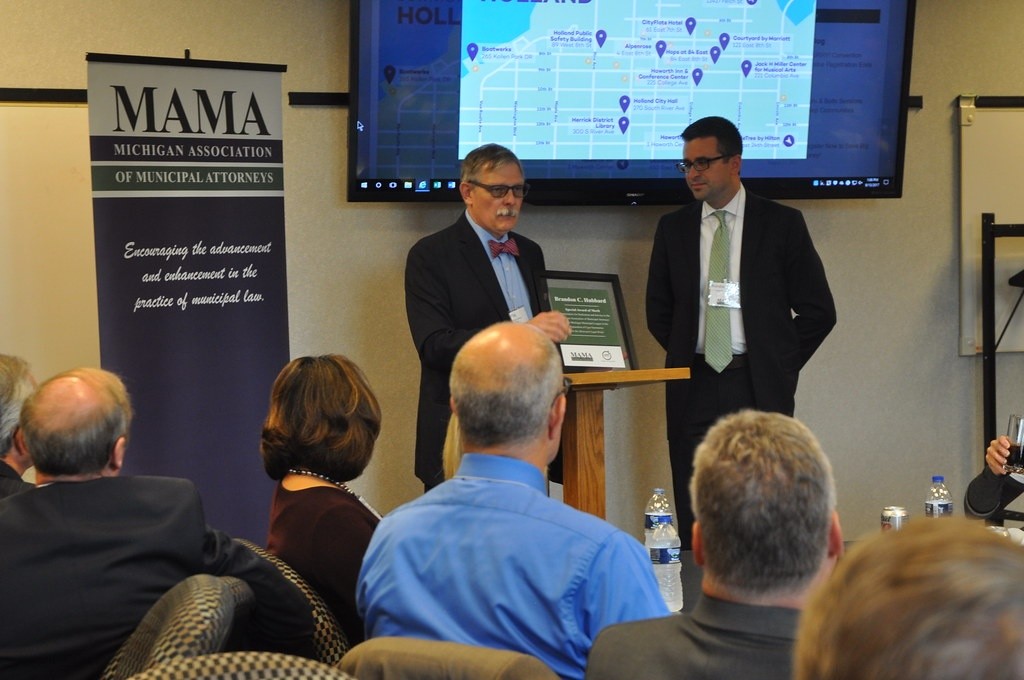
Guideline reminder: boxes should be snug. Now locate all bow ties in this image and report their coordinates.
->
[488,238,520,259]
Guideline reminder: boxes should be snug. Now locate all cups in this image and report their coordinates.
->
[1003,414,1024,474]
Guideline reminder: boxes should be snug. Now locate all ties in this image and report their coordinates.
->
[704,210,734,373]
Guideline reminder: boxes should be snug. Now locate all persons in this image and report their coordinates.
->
[404,143,564,499]
[962,434,1024,520]
[0,356,36,498]
[793,517,1024,680]
[581,410,844,679]
[259,353,385,646]
[645,116,837,551]
[0,368,316,680]
[353,323,671,680]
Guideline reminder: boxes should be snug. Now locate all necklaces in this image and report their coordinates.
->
[289,468,357,498]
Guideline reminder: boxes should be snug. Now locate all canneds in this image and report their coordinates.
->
[880,507,909,532]
[984,525,1011,540]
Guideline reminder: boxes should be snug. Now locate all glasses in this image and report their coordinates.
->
[467,179,531,198]
[675,152,738,173]
[550,377,572,407]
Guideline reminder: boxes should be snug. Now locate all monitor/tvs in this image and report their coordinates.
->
[345,0,917,204]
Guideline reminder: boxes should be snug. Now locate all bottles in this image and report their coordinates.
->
[925,476,953,518]
[644,488,683,613]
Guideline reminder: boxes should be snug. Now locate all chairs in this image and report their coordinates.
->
[99,573,240,679]
[228,536,349,664]
[338,637,560,680]
[132,651,355,680]
